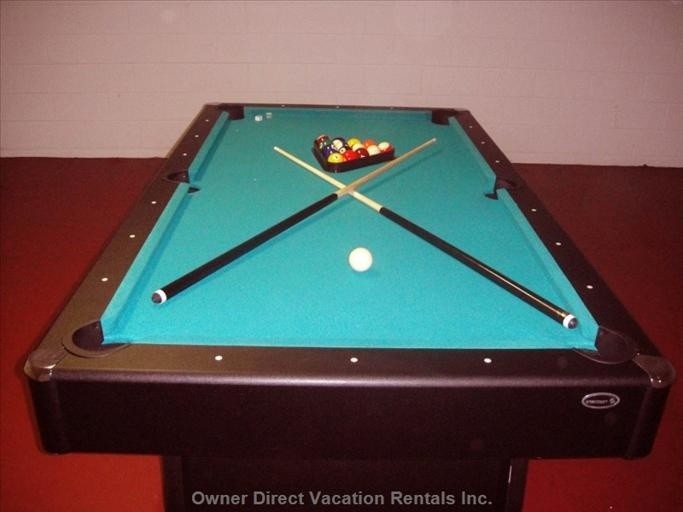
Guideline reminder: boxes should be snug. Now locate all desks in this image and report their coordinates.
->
[23,102,675,512]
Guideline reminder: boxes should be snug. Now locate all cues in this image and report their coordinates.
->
[151,138,436,305]
[273,146,576,329]
